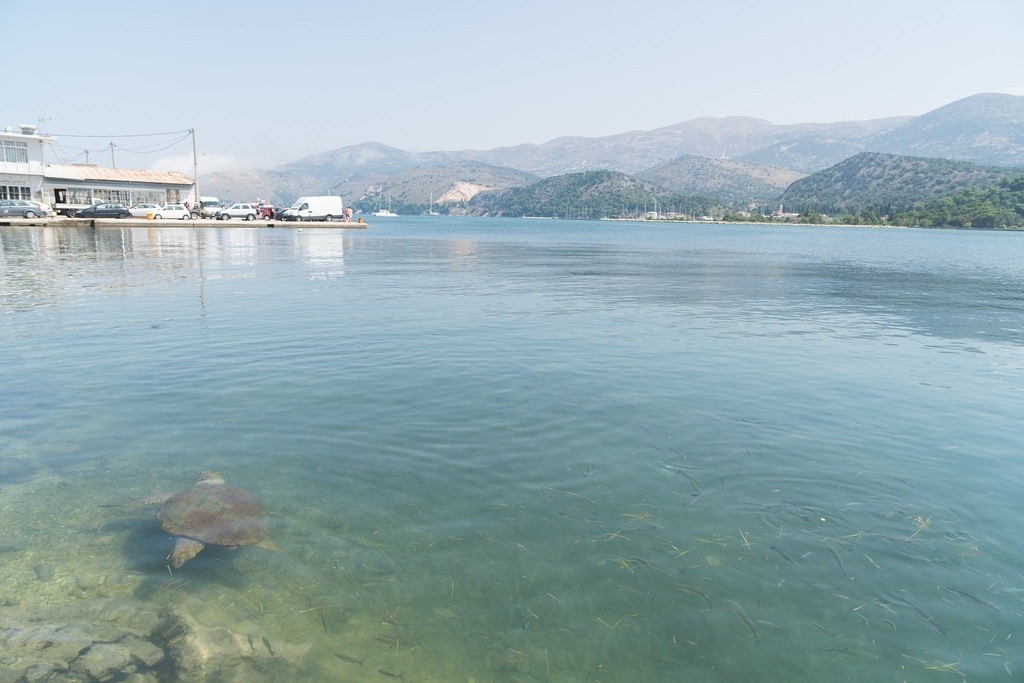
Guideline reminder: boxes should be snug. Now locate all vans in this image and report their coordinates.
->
[281,196,343,222]
[200,196,222,220]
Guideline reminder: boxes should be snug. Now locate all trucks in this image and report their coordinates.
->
[51,197,106,218]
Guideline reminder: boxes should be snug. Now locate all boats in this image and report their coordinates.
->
[374,209,399,218]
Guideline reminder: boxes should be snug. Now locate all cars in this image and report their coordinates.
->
[152,203,191,221]
[80,202,131,220]
[190,196,291,221]
[0,198,56,220]
[127,202,161,220]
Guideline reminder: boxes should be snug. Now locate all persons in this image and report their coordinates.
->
[344,206,353,222]
[184,202,192,213]
[256,206,260,221]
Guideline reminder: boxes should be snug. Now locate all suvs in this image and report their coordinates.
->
[215,203,259,221]
